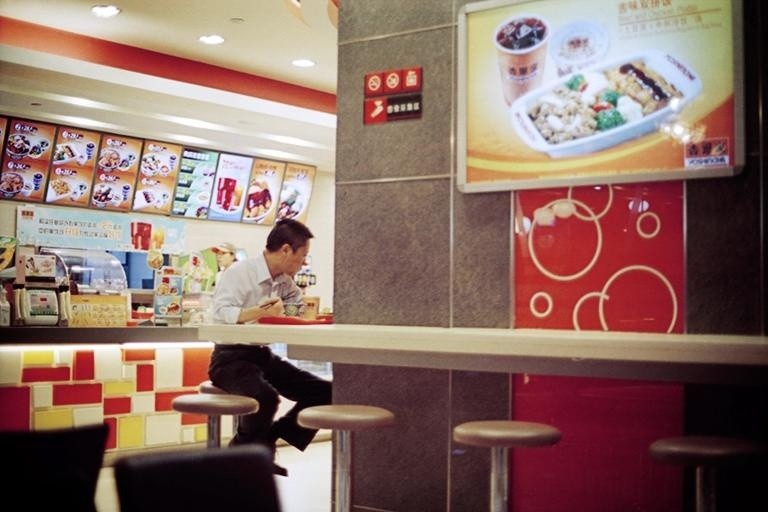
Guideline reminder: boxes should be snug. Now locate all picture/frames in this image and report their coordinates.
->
[457,0,747,195]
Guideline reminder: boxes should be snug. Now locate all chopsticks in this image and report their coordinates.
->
[259,290,297,309]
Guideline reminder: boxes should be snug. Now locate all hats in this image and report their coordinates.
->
[212,242,236,253]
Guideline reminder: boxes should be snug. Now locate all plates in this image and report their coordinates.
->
[509,49,704,159]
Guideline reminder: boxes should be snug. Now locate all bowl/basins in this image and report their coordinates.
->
[0,134,162,209]
[283,303,301,317]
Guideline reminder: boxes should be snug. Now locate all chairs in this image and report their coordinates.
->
[0,422,109,512]
[112,442,283,512]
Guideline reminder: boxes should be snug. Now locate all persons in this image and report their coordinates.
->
[205,219,332,477]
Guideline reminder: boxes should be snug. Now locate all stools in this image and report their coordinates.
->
[199,379,230,394]
[296,405,395,512]
[172,395,260,448]
[650,438,754,512]
[454,420,560,512]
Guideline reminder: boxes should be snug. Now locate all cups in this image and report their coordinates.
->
[160,154,177,177]
[300,295,320,321]
[492,14,610,107]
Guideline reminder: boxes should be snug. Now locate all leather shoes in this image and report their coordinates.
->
[228,433,287,476]
